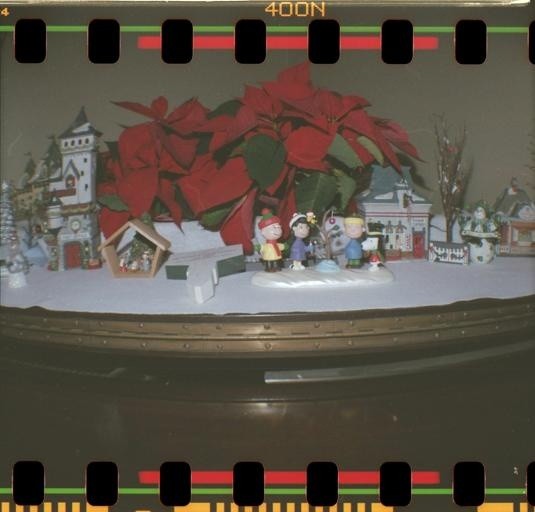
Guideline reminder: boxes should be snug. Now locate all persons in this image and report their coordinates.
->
[257,208,384,274]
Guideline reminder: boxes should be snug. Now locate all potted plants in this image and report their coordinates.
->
[94,96,223,255]
[222,60,434,256]
